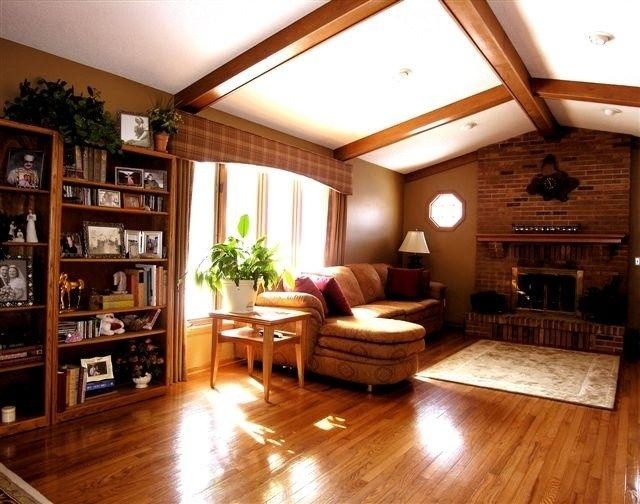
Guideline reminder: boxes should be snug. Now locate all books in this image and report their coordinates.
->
[0,327,45,369]
[57,318,99,340]
[122,262,168,308]
[57,361,89,411]
[138,192,164,211]
[141,309,163,329]
[61,184,91,205]
[66,144,107,182]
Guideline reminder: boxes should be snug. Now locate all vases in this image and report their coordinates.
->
[132,371,152,390]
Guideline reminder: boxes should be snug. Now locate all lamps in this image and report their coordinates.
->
[399,67,411,81]
[466,122,478,131]
[601,108,622,118]
[585,31,615,48]
[398,229,431,270]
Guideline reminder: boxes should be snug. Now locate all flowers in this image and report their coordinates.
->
[115,337,165,378]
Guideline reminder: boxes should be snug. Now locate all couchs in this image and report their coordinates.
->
[234,279,449,393]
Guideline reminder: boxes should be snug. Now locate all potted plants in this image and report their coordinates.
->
[146,94,184,153]
[192,214,294,316]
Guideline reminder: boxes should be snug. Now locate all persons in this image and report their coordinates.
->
[88,362,106,377]
[0,264,9,286]
[24,207,40,243]
[126,171,135,183]
[127,115,149,146]
[103,192,116,206]
[6,150,40,190]
[63,232,83,254]
[146,234,158,254]
[6,264,27,300]
[16,228,23,239]
[8,219,15,241]
[143,172,159,188]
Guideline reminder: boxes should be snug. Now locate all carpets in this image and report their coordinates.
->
[0,463,55,504]
[414,338,620,411]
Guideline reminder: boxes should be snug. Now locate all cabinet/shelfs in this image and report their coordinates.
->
[51,136,178,424]
[0,120,57,438]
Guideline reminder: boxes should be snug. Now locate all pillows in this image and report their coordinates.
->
[253,263,431,322]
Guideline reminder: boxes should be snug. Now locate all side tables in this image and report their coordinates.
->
[211,308,311,403]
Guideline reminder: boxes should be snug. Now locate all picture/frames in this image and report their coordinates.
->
[144,169,168,192]
[0,255,34,307]
[114,166,144,189]
[98,189,122,209]
[80,355,114,382]
[118,112,154,151]
[143,231,162,259]
[82,220,126,260]
[3,147,44,190]
[123,229,141,258]
[122,192,145,210]
